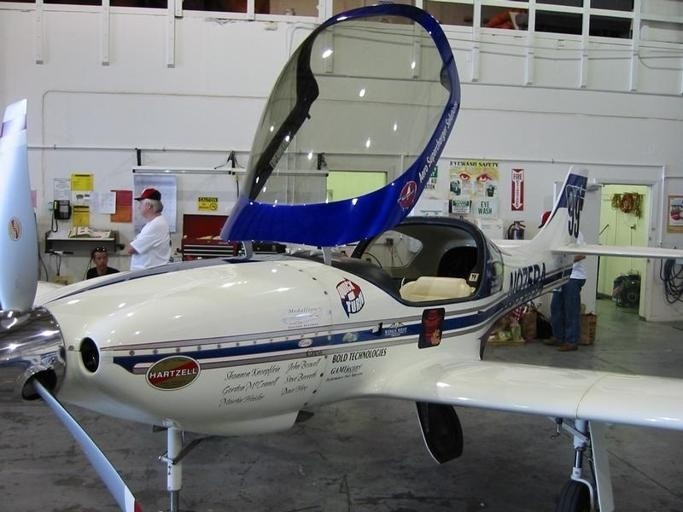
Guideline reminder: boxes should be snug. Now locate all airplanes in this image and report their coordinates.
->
[1,4,683,512]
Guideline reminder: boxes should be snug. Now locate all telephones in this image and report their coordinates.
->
[54,200,71,219]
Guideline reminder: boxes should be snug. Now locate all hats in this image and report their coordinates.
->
[134,188,161,201]
[538,211,552,228]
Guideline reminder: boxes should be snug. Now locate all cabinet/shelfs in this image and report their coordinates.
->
[46,229,119,253]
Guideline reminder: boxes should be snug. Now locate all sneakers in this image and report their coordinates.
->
[558,343,578,352]
[542,337,560,346]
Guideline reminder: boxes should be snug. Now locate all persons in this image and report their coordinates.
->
[458,166,493,197]
[537,208,587,353]
[85,246,120,280]
[125,186,173,273]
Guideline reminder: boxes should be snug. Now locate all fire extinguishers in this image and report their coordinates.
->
[508,221,526,240]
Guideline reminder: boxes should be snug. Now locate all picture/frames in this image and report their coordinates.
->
[667,195,683,233]
